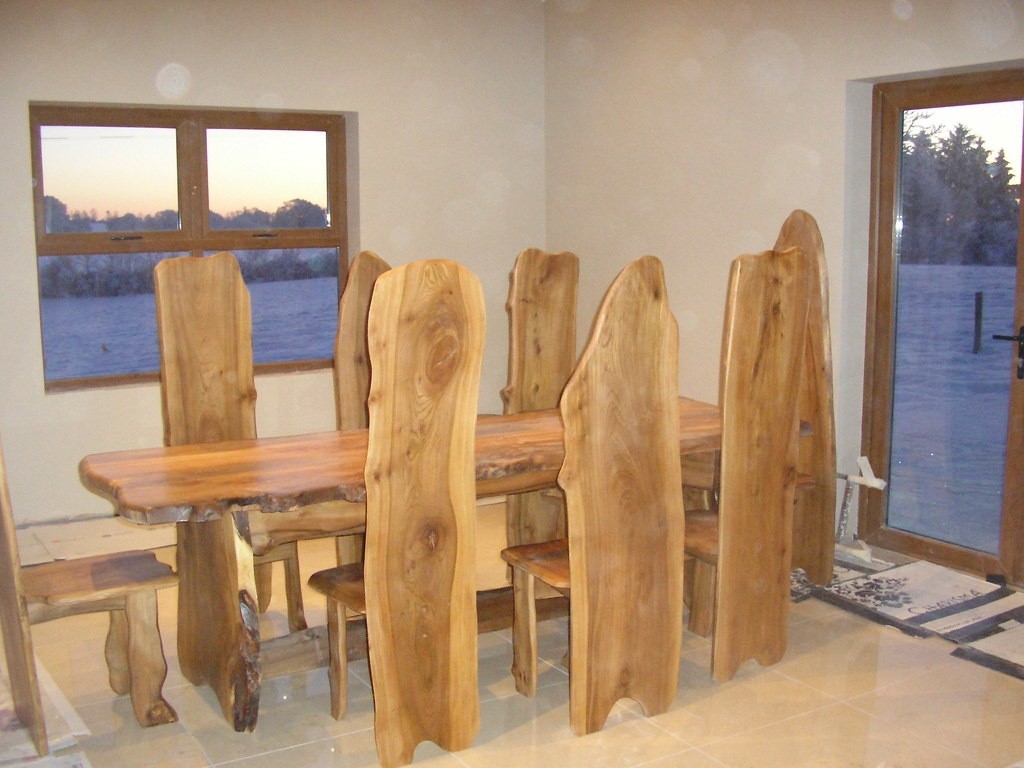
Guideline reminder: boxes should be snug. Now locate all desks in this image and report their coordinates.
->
[78,395,814,734]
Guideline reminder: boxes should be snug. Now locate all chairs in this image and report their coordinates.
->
[683,246,810,682]
[306,260,491,768]
[155,253,309,634]
[333,249,395,626]
[772,210,835,587]
[499,255,684,735]
[501,247,579,589]
[0,439,181,755]
